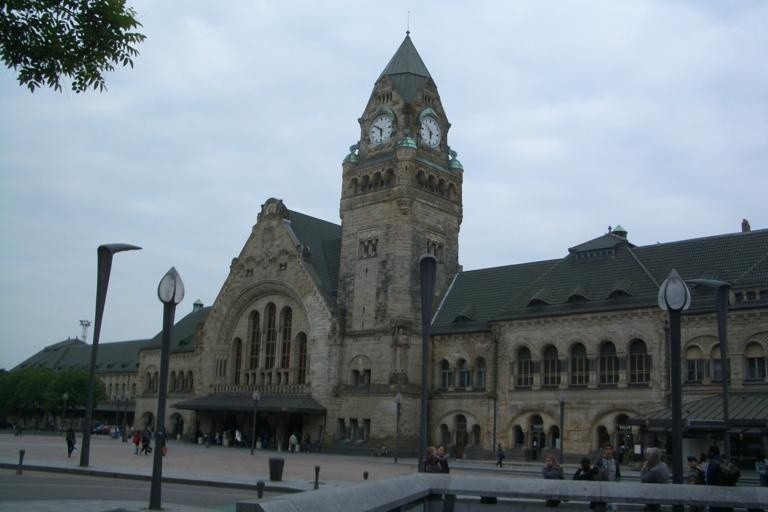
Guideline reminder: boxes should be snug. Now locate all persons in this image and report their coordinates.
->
[215,426,243,448]
[163,427,169,456]
[571,441,620,512]
[495,443,505,468]
[304,433,312,454]
[91,424,154,456]
[12,422,19,436]
[288,433,300,453]
[753,447,767,487]
[687,444,740,512]
[422,444,449,500]
[542,455,564,507]
[639,447,669,511]
[65,428,76,458]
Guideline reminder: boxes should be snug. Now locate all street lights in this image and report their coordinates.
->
[250,391,260,454]
[112,391,129,442]
[150,266,186,510]
[394,392,402,463]
[658,269,691,512]
[61,392,69,435]
[558,391,566,464]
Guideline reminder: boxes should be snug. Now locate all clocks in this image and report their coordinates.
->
[420,115,441,149]
[367,113,394,145]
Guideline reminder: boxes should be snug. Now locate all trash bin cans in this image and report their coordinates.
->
[176,434,181,441]
[198,437,203,445]
[256,442,262,449]
[269,459,284,481]
[524,449,537,461]
